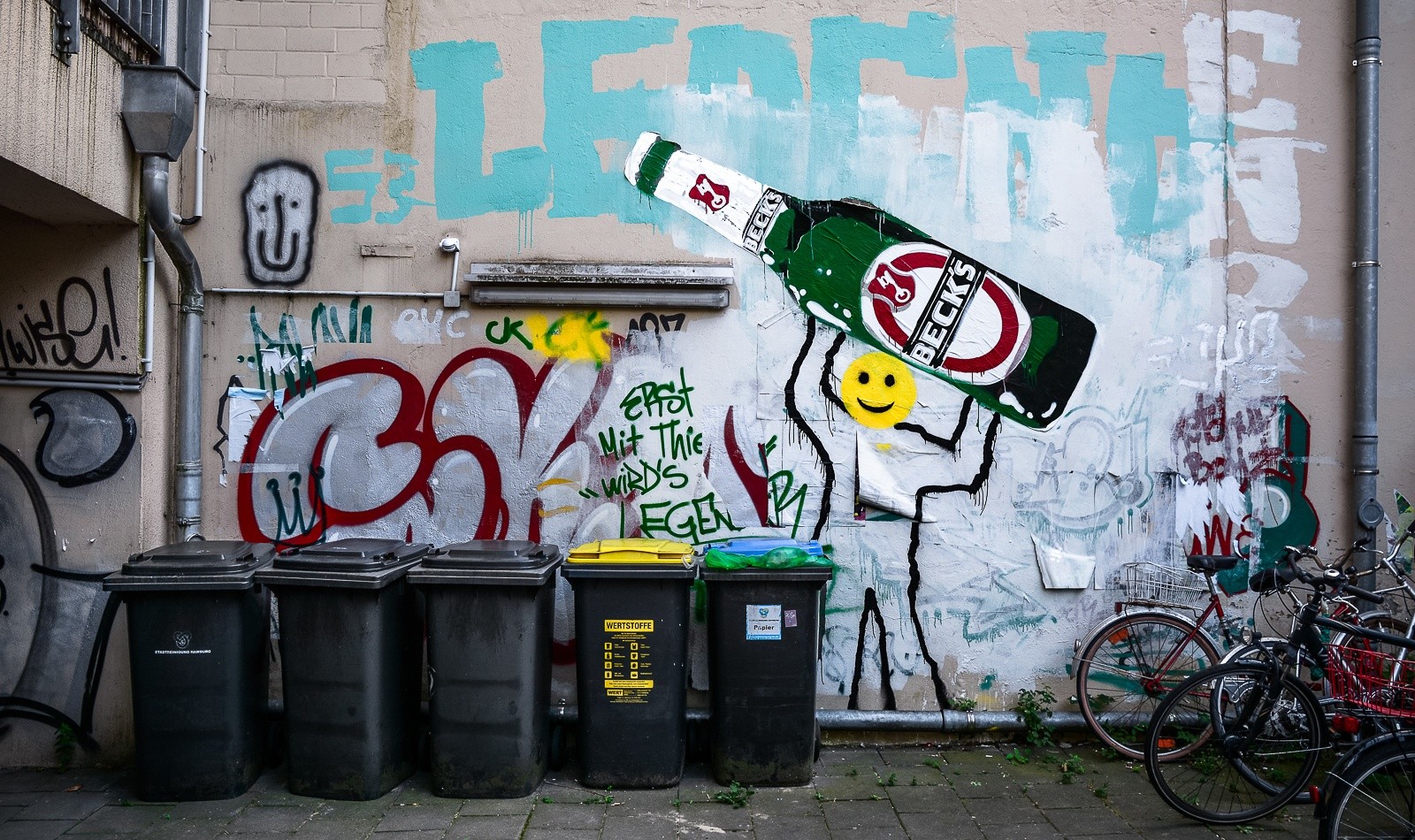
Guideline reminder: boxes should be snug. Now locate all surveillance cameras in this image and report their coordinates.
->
[442,239,457,252]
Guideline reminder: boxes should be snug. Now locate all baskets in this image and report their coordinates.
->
[1325,643,1415,718]
[1121,561,1203,607]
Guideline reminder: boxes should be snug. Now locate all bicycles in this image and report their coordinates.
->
[1070,520,1415,839]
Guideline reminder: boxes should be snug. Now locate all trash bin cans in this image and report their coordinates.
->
[698,539,834,788]
[561,538,699,791]
[255,536,434,801]
[406,538,565,799]
[101,539,278,801]
